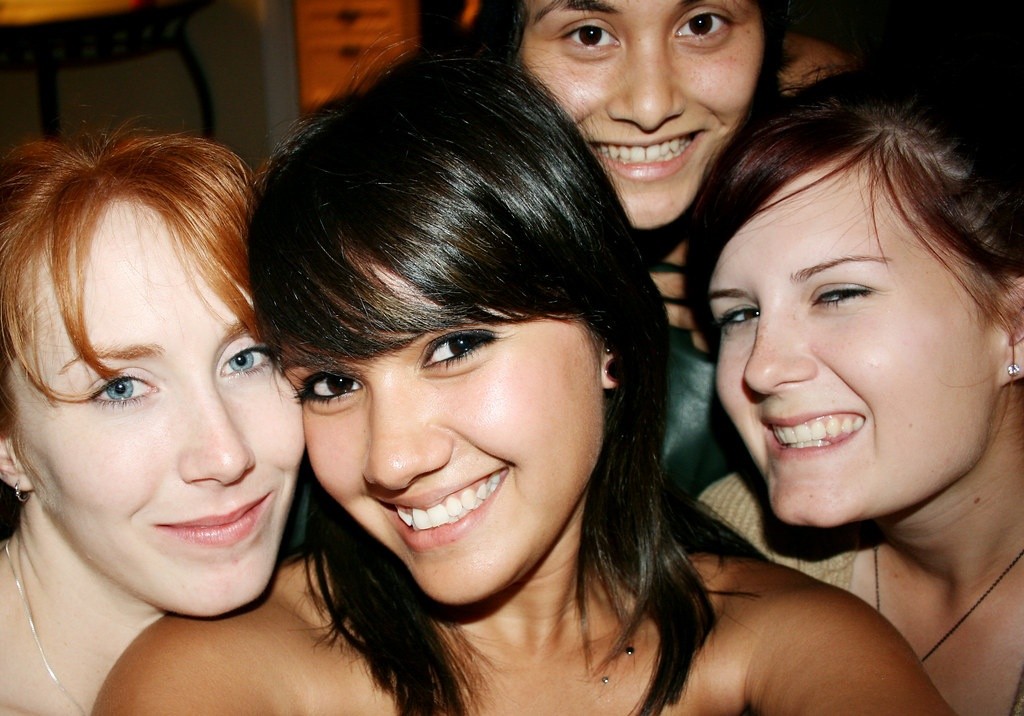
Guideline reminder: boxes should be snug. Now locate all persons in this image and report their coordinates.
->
[89,37,959,716]
[0,115,308,716]
[465,0,1024,716]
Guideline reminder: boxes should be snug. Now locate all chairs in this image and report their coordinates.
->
[0,0,217,142]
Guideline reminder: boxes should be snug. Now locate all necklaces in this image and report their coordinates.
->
[873,531,1024,664]
[5,538,87,716]
